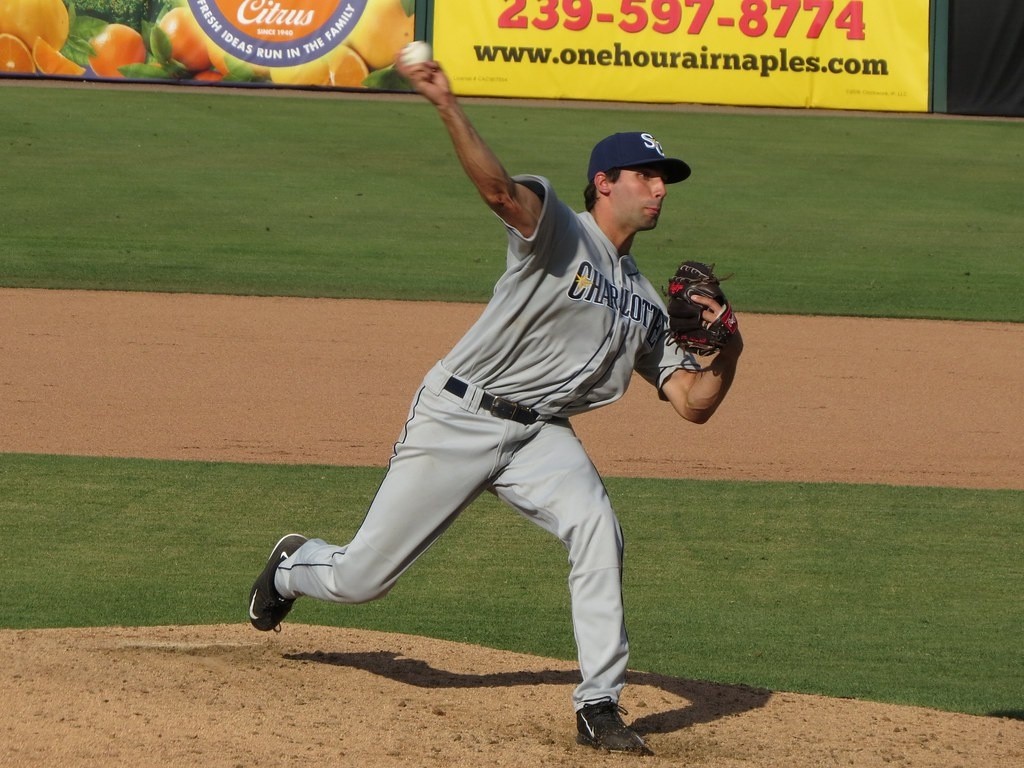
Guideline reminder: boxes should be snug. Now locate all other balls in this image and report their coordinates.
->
[402,37,435,73]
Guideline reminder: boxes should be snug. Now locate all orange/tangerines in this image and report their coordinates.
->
[0,0,414,88]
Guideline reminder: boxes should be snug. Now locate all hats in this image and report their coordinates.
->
[587,131,692,183]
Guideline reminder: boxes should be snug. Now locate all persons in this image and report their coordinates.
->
[245,50,743,756]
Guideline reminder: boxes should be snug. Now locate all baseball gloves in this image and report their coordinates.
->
[657,257,738,359]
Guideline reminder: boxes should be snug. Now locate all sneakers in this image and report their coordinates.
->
[577,703,649,754]
[249,534,309,632]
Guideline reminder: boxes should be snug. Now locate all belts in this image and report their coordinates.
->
[444,377,539,425]
